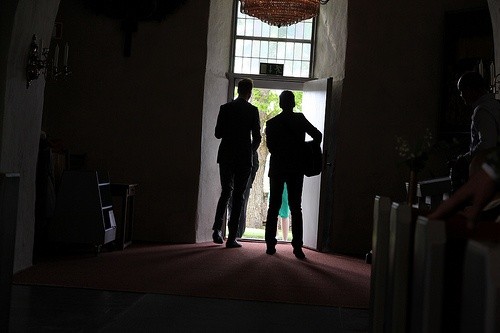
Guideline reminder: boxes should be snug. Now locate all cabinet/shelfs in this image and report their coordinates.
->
[55,171,117,255]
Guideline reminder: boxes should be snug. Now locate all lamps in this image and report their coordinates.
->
[240,0,329,28]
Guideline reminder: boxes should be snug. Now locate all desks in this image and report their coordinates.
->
[112,182,137,249]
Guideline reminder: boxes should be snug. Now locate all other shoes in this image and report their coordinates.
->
[293,247,306,259]
[226,238,242,248]
[212,229,223,244]
[266,245,276,255]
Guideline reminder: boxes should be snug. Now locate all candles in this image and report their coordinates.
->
[54,43,59,68]
[63,42,69,67]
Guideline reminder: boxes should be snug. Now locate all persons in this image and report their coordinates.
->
[426,70,500,230]
[266,91,321,259]
[213,79,261,248]
